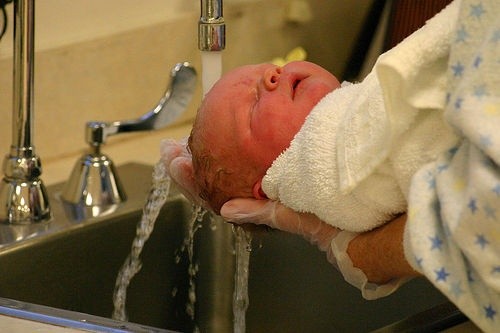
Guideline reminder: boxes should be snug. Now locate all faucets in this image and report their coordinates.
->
[0,0,228,228]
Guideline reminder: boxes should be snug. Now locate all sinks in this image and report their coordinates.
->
[0,153,473,332]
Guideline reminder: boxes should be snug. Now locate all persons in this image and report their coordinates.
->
[163,0,499,333]
[185,60,415,234]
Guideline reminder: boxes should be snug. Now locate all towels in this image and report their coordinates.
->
[259,0,462,227]
